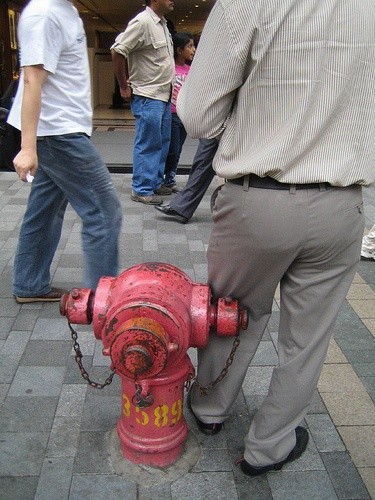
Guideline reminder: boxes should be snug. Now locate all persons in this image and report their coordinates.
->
[361,223,375,261]
[154,139,219,224]
[110,1,175,206]
[176,1,375,477]
[163,33,196,193]
[6,0,123,303]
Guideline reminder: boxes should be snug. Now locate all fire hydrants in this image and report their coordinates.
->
[60,262,249,469]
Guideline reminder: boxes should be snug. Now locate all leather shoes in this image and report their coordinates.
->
[154,205,189,224]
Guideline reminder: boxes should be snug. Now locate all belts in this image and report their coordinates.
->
[227,174,329,190]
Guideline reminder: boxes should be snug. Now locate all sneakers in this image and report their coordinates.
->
[154,186,172,195]
[131,191,163,205]
[360,250,375,260]
[171,185,184,194]
[234,426,309,476]
[14,287,68,304]
[187,382,223,434]
